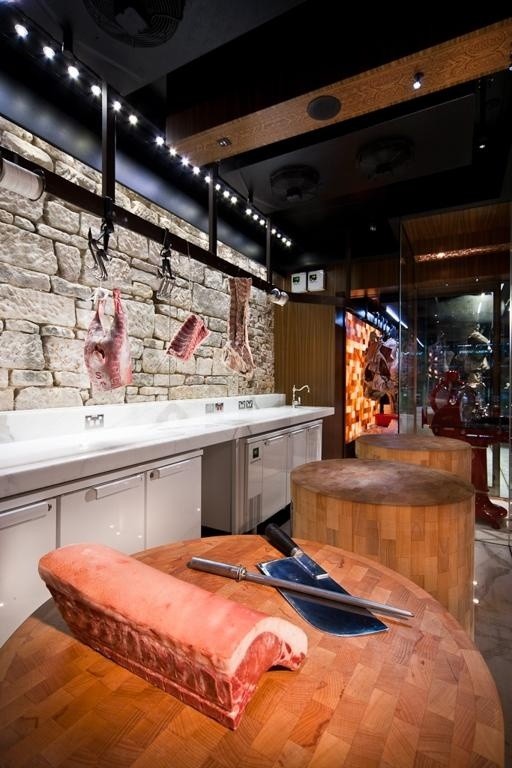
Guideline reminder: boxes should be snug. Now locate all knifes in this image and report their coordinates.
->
[259,521,388,638]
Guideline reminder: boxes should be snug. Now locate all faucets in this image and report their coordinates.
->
[292,385,310,408]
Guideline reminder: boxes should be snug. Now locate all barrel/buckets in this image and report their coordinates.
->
[399,414,414,434]
[373,413,398,428]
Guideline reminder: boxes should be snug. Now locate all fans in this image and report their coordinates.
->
[92,1,184,50]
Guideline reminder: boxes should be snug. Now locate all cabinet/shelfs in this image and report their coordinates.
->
[1,485,59,645]
[56,450,204,593]
[207,418,325,534]
